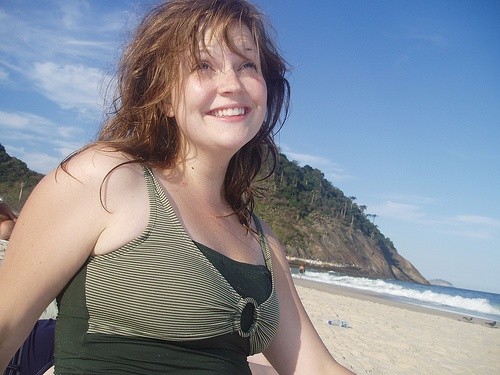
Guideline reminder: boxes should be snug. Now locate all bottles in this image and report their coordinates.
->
[328,320,350,328]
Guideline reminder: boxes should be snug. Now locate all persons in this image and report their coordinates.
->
[1,0,357,375]
[0,202,19,240]
